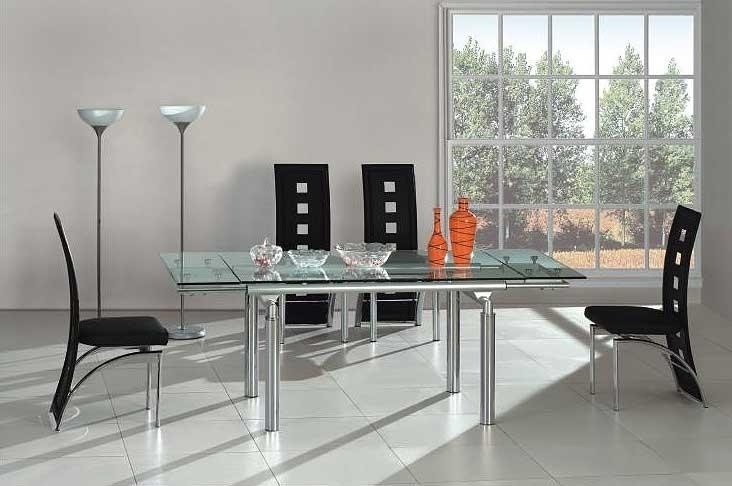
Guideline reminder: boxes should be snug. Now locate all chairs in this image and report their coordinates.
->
[586,204,711,411]
[46,211,168,434]
[273,162,442,345]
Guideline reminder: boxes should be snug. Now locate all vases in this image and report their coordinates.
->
[427,197,477,266]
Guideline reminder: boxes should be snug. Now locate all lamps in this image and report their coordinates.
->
[75,105,208,341]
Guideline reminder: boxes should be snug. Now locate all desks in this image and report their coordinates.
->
[158,248,589,432]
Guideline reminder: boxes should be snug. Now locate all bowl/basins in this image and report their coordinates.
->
[288,249,330,268]
[335,242,393,269]
